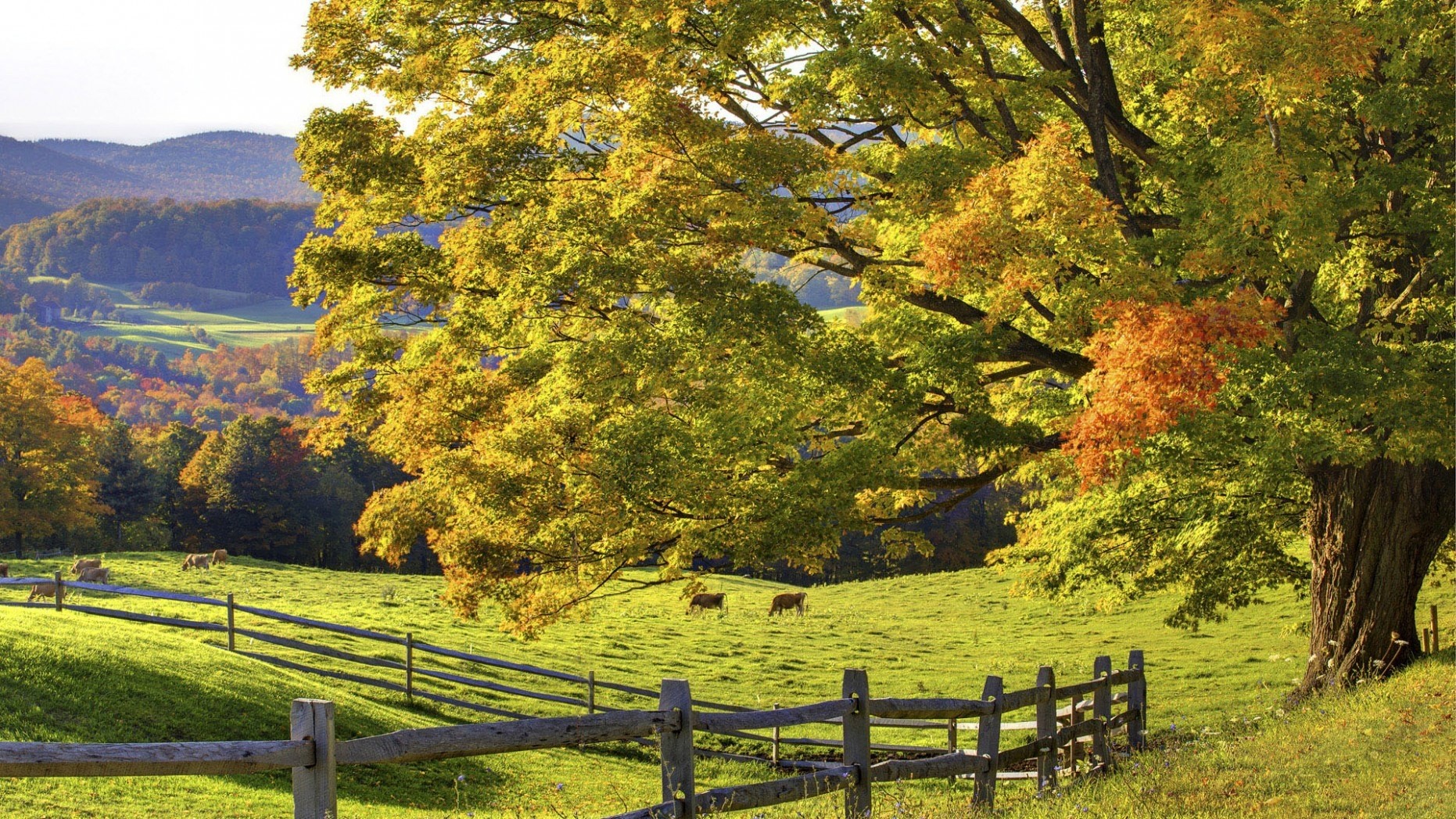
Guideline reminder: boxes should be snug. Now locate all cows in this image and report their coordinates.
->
[767,593,810,618]
[0,562,12,579]
[72,559,102,574]
[212,549,228,565]
[75,567,110,584]
[686,592,729,616]
[27,579,68,603]
[182,553,209,570]
